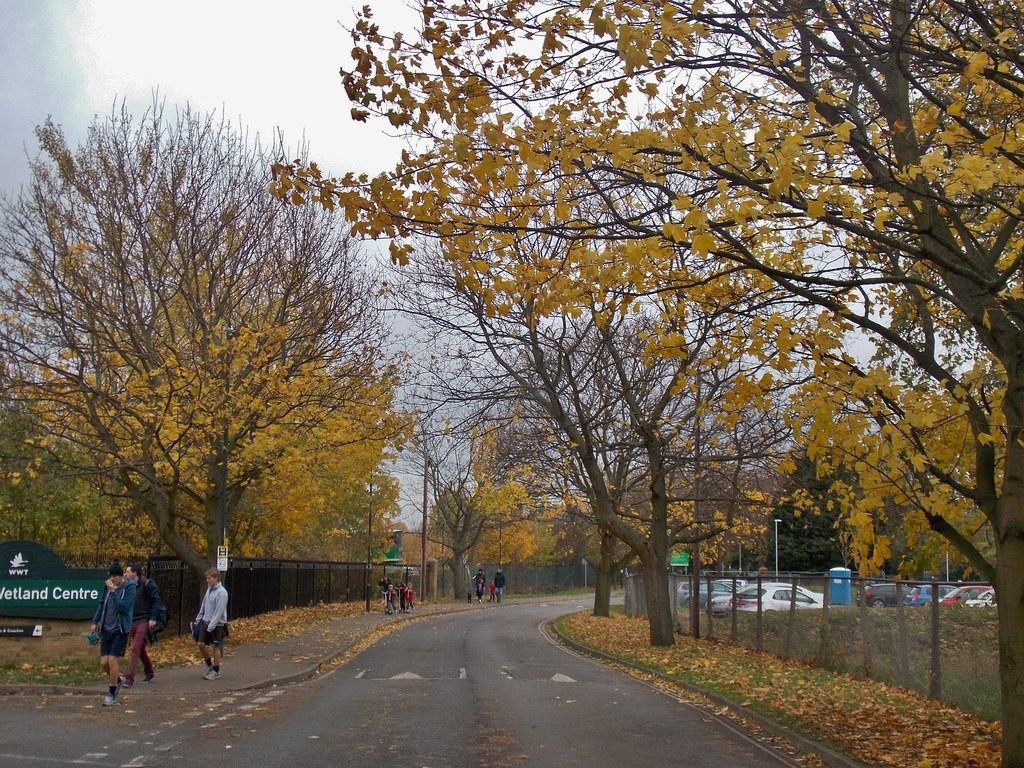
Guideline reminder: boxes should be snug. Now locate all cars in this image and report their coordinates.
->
[966,590,997,610]
[676,579,824,617]
[930,585,993,609]
[904,585,968,607]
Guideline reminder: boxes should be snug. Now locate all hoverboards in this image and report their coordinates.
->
[383,587,411,615]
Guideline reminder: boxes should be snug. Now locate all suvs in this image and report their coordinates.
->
[856,583,912,608]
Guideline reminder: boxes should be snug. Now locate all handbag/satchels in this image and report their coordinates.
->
[148,601,169,632]
[191,621,228,642]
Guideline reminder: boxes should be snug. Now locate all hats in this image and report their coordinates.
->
[107,561,123,576]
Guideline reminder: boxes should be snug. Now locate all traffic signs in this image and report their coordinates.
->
[0,625,43,637]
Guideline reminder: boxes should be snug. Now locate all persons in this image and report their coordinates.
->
[472,568,486,603]
[384,584,397,615]
[193,567,229,680]
[493,566,505,603]
[394,579,415,614]
[90,558,140,707]
[377,576,393,608]
[487,581,496,602]
[118,565,162,687]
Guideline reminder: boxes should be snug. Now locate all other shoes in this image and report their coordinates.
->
[116,677,123,694]
[141,675,153,684]
[123,681,132,688]
[102,694,115,706]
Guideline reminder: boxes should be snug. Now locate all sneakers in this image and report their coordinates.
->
[203,665,221,680]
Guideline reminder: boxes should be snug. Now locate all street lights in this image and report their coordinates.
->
[774,520,782,596]
